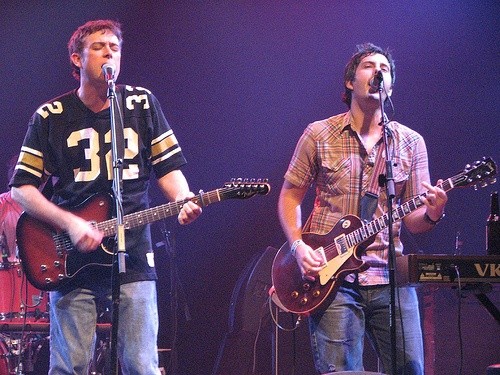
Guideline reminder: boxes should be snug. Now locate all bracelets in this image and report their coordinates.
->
[290,239,306,256]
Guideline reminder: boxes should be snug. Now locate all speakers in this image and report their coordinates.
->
[209,245,307,374]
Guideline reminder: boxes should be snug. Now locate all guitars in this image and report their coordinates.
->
[17,178,271,291]
[271,156,497,316]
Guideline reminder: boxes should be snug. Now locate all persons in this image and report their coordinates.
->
[0,155,47,264]
[278,43,448,375]
[9,19,202,375]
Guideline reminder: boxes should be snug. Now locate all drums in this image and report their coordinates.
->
[21,335,106,375]
[0,261,49,319]
[0,333,23,375]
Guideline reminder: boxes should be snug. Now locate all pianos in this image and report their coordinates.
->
[395,255,500,326]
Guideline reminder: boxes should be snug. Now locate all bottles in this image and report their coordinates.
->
[452,232,463,256]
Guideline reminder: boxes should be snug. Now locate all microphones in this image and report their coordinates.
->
[102,62,117,99]
[370,70,383,87]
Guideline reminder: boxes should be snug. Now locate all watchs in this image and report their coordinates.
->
[424,212,446,225]
